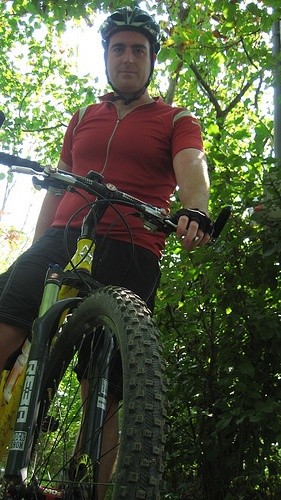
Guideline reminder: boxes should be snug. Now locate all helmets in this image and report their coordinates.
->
[99,6,162,54]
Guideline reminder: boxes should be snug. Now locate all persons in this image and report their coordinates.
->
[0,6,223,499]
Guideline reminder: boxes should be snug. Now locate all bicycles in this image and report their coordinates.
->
[0,109,233,500]
